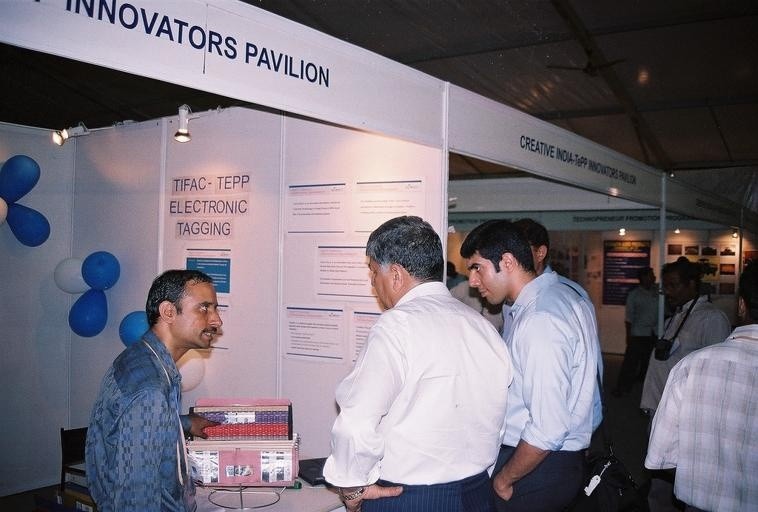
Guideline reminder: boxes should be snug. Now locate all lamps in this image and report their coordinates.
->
[51,122,88,146]
[174,103,193,143]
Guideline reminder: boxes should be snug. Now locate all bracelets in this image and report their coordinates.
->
[338,487,365,501]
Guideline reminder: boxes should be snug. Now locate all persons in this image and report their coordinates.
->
[614,265,660,397]
[84,269,223,512]
[321,215,515,512]
[447,259,505,333]
[639,261,732,436]
[459,216,598,512]
[644,261,758,512]
[513,217,605,512]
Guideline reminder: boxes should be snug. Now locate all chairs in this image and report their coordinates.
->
[58,427,97,511]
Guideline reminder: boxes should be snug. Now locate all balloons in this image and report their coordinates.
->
[54,251,121,338]
[117,311,147,347]
[0,155,51,246]
[175,349,205,391]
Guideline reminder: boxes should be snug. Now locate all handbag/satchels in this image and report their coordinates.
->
[576,451,652,512]
